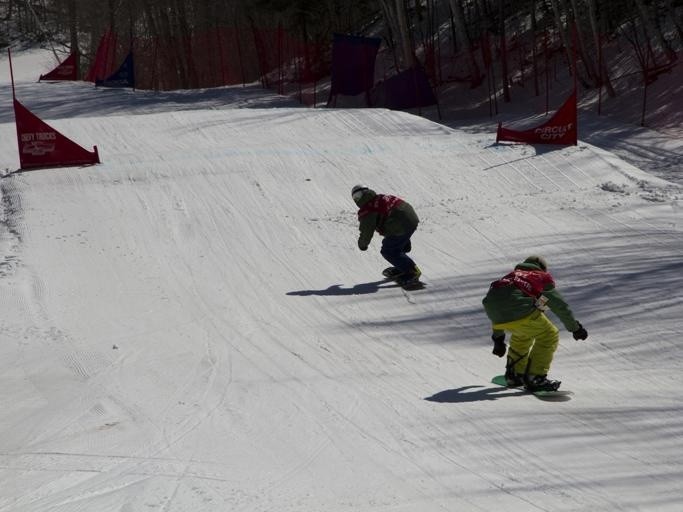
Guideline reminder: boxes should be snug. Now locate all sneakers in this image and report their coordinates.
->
[400,265,421,284]
[523,374,561,391]
[504,372,525,387]
[388,267,404,277]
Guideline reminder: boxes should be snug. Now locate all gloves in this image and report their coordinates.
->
[572,320,588,341]
[492,333,506,357]
[357,238,370,251]
[405,240,412,253]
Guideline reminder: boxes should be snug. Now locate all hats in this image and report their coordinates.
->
[351,184,369,194]
[525,255,548,272]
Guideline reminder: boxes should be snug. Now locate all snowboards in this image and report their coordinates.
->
[383,267,427,290]
[491,375,575,396]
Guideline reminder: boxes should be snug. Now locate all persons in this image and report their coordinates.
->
[351,183,422,285]
[481,255,590,392]
